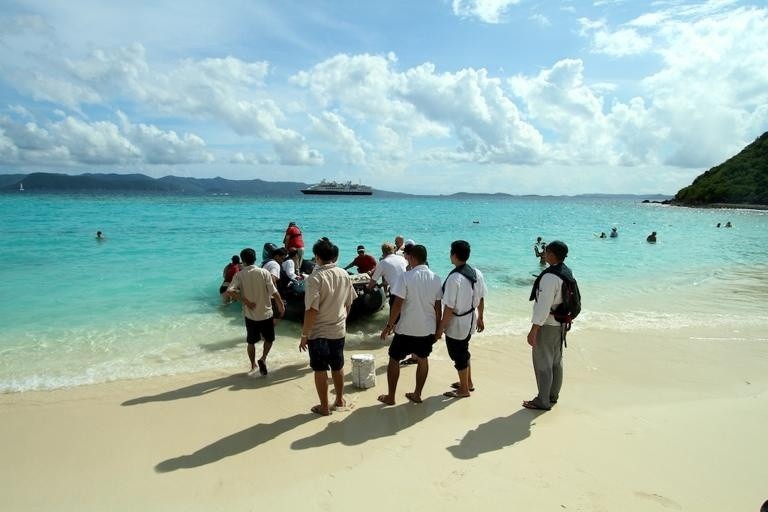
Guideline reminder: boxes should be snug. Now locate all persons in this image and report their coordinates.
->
[299,238,357,412]
[440,241,486,398]
[610,226,618,236]
[648,231,657,241]
[534,244,546,263]
[219,222,379,320]
[225,248,286,374]
[400,243,428,272]
[369,242,409,334]
[378,247,442,404]
[393,235,409,259]
[522,242,577,409]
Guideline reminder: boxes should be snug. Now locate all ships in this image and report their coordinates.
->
[300,178,374,196]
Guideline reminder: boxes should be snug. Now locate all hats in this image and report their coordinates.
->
[357,246,364,251]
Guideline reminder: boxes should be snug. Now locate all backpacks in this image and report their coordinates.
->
[553,277,581,323]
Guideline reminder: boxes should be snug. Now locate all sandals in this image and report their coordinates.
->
[401,358,418,365]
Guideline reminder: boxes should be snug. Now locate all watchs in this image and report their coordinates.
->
[385,322,393,329]
[300,333,308,338]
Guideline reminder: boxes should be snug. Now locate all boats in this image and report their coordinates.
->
[263,243,386,324]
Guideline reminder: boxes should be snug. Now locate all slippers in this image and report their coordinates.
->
[405,393,422,404]
[258,360,268,376]
[523,398,557,410]
[444,382,475,397]
[311,399,346,415]
[378,394,395,406]
